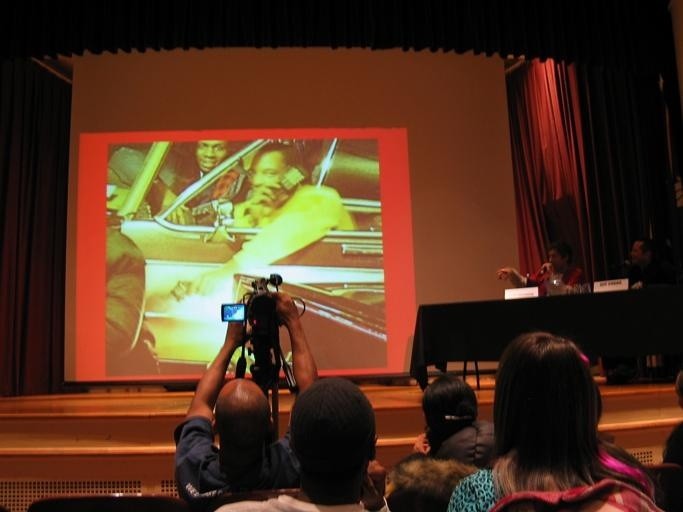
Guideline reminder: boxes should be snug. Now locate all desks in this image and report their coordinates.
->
[419,284,683,390]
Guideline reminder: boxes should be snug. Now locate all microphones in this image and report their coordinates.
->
[538,266,547,276]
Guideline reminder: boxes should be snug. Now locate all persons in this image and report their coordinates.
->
[662,371,683,467]
[444,330,653,511]
[174,290,320,500]
[101,139,363,378]
[595,383,662,511]
[215,379,389,511]
[618,236,668,289]
[494,243,584,294]
[369,375,496,512]
